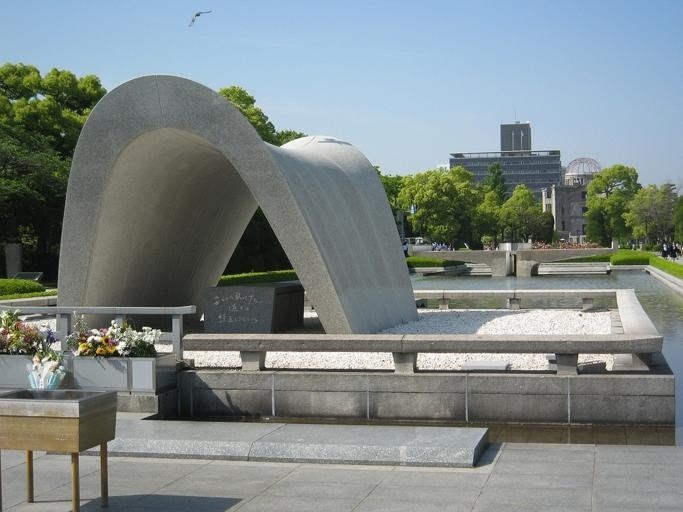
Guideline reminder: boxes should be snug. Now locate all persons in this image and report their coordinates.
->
[430,241,456,252]
[659,239,683,262]
[401,239,408,257]
